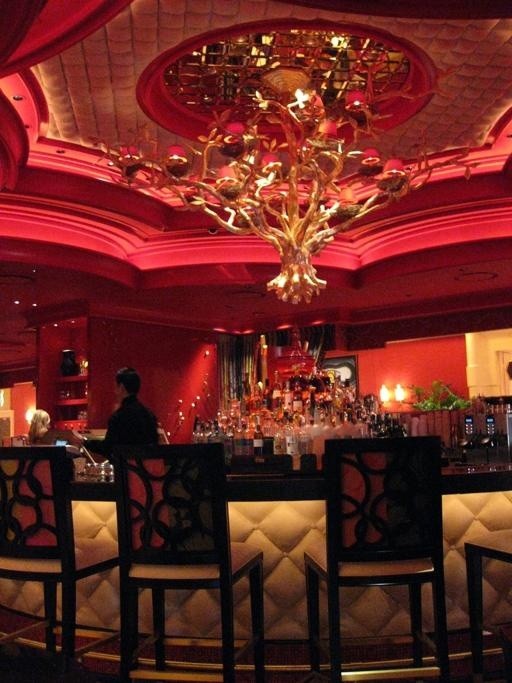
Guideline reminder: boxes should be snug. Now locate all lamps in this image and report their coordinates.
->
[109,58,491,304]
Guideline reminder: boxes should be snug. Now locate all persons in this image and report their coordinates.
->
[27,409,51,446]
[72,366,162,468]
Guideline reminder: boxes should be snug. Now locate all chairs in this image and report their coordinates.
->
[2,445,119,682]
[113,442,266,683]
[303,436,446,681]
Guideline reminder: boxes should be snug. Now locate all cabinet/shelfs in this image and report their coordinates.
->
[36,313,91,425]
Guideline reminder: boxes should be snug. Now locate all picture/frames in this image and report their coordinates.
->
[320,354,360,399]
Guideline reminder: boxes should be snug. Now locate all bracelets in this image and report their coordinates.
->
[81,437,88,444]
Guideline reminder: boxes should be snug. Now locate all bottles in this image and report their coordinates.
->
[192,369,401,470]
